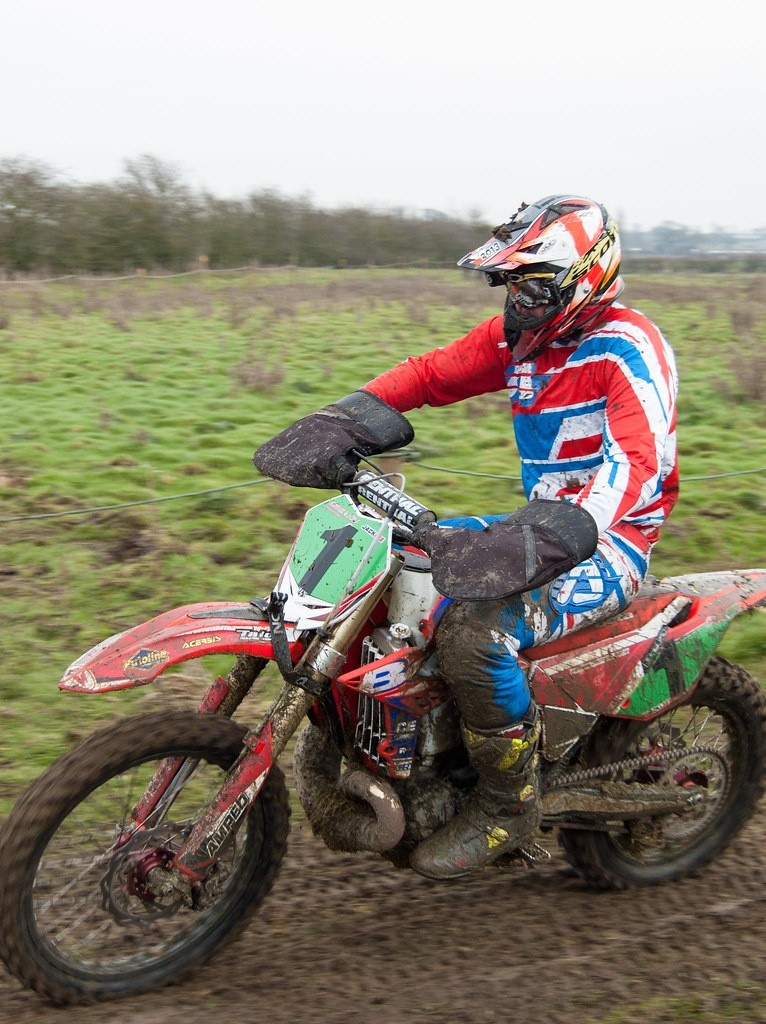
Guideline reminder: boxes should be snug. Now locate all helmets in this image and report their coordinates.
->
[456,194,625,362]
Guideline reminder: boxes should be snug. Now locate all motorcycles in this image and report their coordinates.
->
[0,447,766,1004]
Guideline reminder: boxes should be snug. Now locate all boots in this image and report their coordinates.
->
[408,696,543,880]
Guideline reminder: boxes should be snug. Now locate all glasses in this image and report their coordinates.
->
[507,280,561,308]
[496,271,556,283]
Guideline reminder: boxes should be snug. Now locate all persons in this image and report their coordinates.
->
[252,194,679,882]
